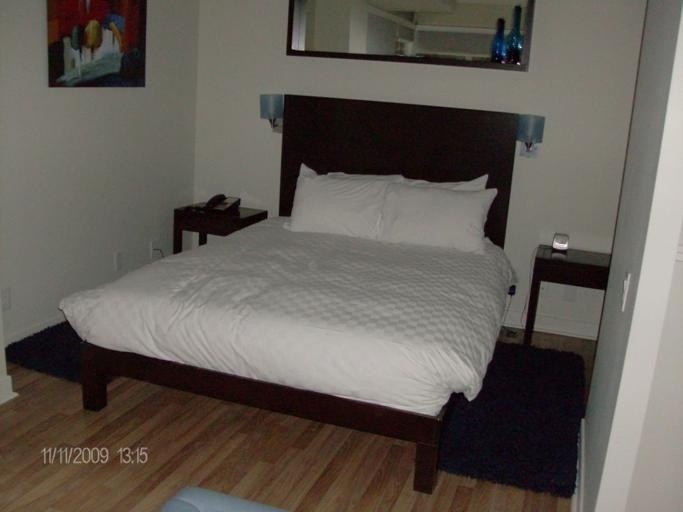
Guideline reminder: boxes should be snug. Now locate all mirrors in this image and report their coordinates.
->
[286,0,535,72]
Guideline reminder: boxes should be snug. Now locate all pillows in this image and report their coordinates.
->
[285,163,498,254]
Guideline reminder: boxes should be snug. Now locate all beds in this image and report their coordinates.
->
[58,95,518,495]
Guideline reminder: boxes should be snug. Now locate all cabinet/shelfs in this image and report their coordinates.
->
[412,22,525,62]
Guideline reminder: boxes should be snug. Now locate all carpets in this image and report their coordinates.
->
[436,341,585,498]
[6,320,83,383]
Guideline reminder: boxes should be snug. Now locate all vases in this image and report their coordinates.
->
[491,17,512,62]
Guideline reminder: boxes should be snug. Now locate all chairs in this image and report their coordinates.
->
[160,486,290,512]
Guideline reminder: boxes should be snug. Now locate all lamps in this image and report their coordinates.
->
[260,94,284,130]
[518,115,545,155]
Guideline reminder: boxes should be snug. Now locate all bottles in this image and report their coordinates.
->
[491,18,506,64]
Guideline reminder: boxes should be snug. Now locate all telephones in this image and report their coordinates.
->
[202,194,240,217]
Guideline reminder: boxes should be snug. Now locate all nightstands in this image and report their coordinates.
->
[173,203,268,253]
[524,245,610,345]
